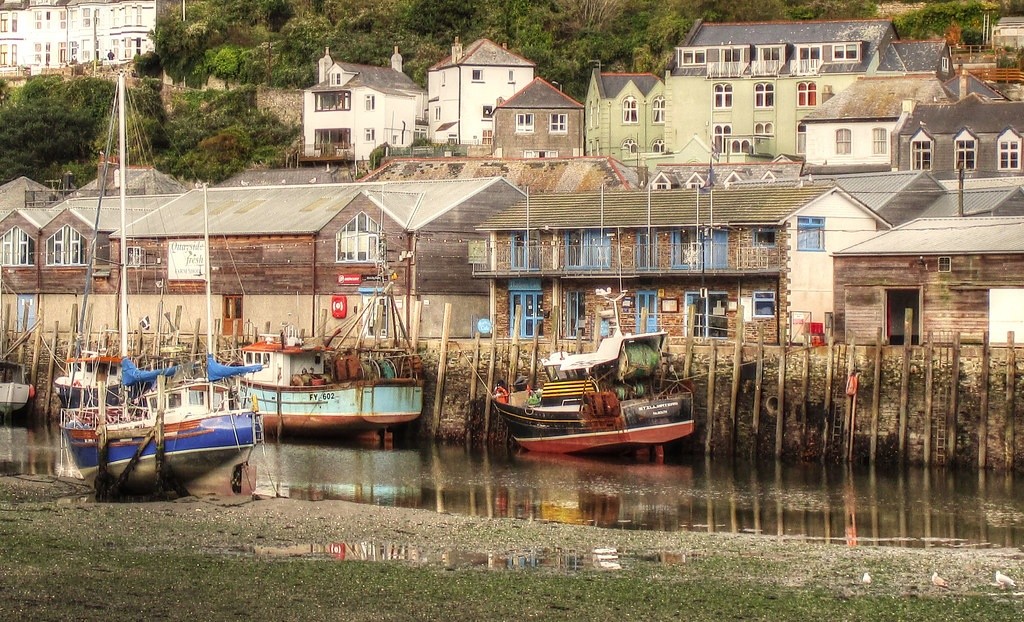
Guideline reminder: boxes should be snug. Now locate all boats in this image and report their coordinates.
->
[0,359,31,422]
[53,68,426,503]
[488,287,697,465]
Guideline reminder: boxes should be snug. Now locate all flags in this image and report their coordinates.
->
[139,316,150,330]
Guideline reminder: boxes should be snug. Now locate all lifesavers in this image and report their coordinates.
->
[253,393,260,413]
[494,387,509,404]
